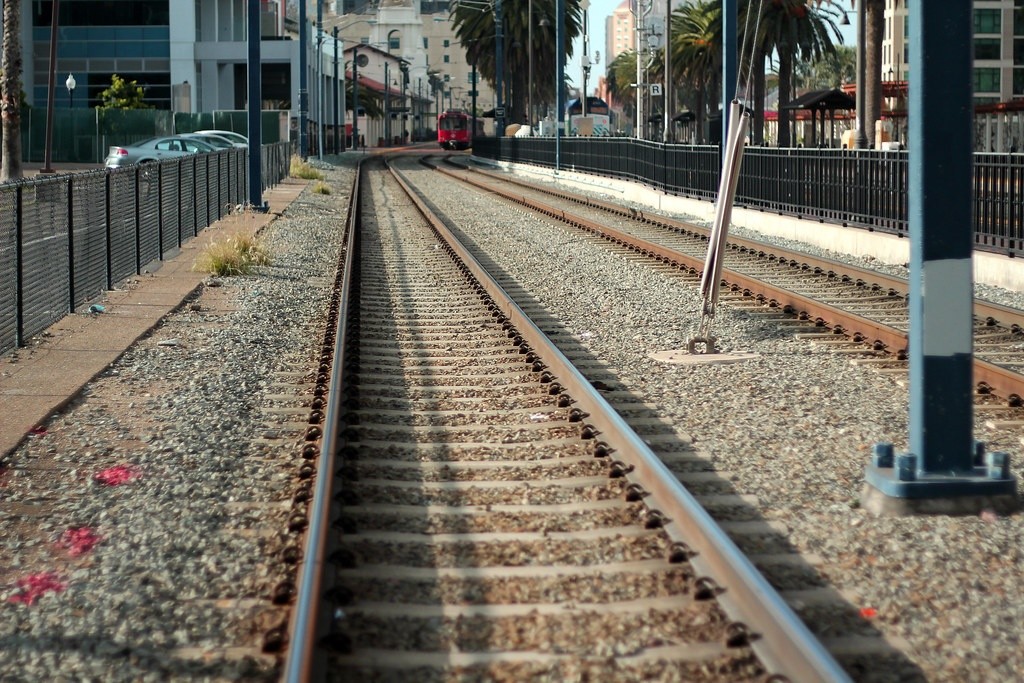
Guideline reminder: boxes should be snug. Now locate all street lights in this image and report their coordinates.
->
[333,20,377,155]
[578,0,591,117]
[319,38,344,167]
[385,56,414,148]
[353,42,388,151]
[434,18,476,156]
[66,73,77,163]
[529,0,550,136]
[403,65,429,146]
[419,74,463,141]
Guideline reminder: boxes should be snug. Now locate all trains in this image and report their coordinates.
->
[437,109,485,150]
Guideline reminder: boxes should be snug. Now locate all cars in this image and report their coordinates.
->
[104,129,265,182]
